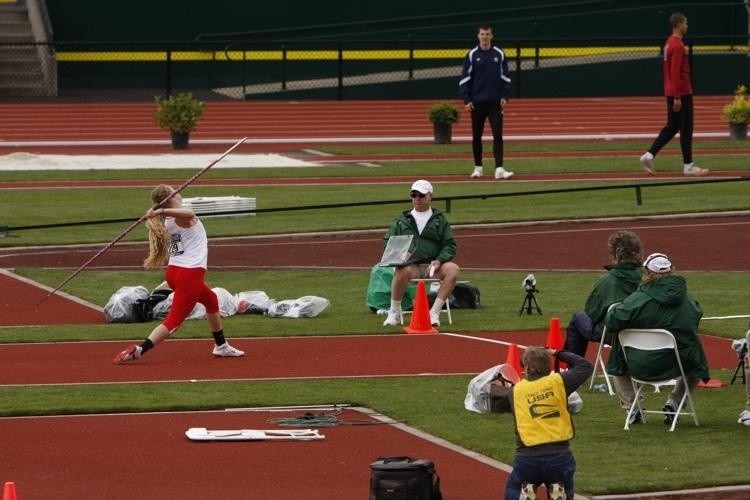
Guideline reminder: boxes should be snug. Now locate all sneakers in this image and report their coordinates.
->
[548,481,568,500]
[737,410,750,426]
[640,152,656,176]
[471,166,483,178]
[683,162,709,176]
[429,310,440,327]
[624,408,646,425]
[662,403,680,426]
[518,480,538,500]
[383,312,403,327]
[495,167,514,179]
[212,340,245,357]
[113,345,143,363]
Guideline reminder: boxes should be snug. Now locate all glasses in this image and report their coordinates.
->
[411,194,425,198]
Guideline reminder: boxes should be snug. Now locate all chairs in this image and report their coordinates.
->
[587,302,625,396]
[618,329,704,433]
[393,267,453,324]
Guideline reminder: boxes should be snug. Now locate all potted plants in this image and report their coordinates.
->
[720,86,750,140]
[152,90,205,149]
[426,100,463,145]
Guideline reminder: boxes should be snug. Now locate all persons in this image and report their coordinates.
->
[604,251,710,427]
[640,12,712,175]
[502,344,595,500]
[559,230,646,359]
[381,180,461,328]
[113,183,246,364]
[456,21,518,180]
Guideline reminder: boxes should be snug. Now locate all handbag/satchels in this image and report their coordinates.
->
[369,456,441,500]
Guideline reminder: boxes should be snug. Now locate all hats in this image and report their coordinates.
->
[642,253,672,273]
[410,180,433,195]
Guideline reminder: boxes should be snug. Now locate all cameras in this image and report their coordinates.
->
[732,339,747,358]
[525,278,541,293]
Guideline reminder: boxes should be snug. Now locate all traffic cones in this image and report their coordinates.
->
[546,316,566,370]
[403,279,439,334]
[504,342,523,384]
[2,481,20,500]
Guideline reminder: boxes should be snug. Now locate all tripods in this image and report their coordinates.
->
[518,293,542,316]
[731,358,746,386]
